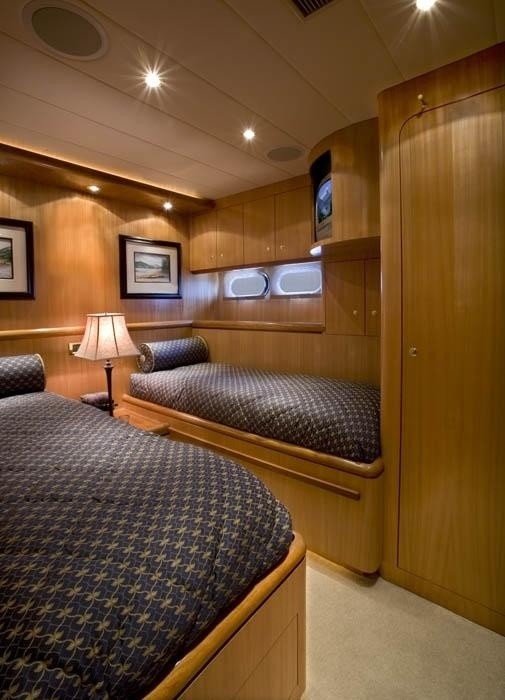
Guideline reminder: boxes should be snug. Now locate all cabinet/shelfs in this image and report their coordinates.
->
[245,185,314,263]
[186,201,244,271]
[325,257,384,338]
[393,87,505,620]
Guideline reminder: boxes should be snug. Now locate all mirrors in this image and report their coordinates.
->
[0,216,38,302]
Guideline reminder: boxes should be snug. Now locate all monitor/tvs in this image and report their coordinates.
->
[314,174,332,240]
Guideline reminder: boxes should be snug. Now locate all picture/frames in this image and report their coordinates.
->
[117,232,184,302]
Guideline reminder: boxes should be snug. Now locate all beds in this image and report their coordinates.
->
[1,390,307,699]
[119,361,389,576]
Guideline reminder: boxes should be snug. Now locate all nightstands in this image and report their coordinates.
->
[107,406,168,437]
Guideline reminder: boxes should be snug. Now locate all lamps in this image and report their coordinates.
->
[72,311,141,420]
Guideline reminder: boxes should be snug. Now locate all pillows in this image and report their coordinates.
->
[137,334,208,374]
[1,351,48,399]
